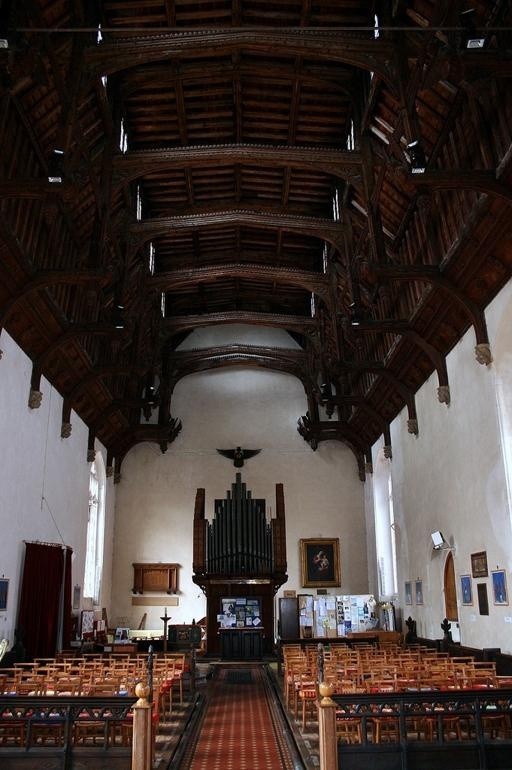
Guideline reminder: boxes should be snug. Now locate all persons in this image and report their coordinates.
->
[302,626,312,638]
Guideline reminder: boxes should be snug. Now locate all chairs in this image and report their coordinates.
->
[0,651,193,750]
[281,640,509,767]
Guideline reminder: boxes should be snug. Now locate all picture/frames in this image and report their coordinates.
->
[458,550,509,617]
[299,537,341,588]
